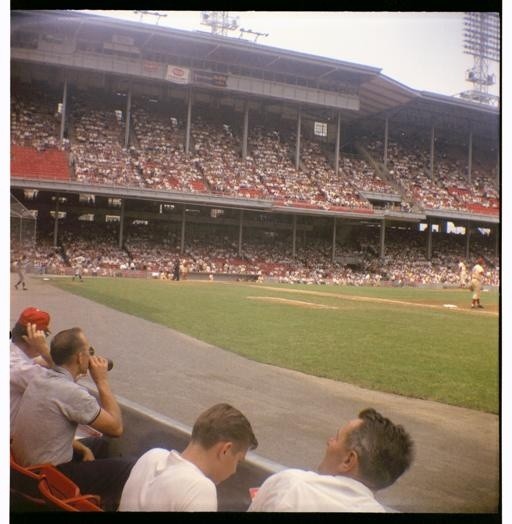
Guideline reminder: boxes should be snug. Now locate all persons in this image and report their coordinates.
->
[471,259,488,309]
[10,307,55,437]
[13,328,135,511]
[247,408,415,514]
[117,402,258,513]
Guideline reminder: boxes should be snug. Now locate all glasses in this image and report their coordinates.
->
[82,347,95,356]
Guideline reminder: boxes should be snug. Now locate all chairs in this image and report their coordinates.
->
[11,451,107,513]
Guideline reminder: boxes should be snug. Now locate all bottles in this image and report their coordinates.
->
[87,347,113,373]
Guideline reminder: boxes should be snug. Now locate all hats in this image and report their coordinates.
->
[17,307,52,335]
